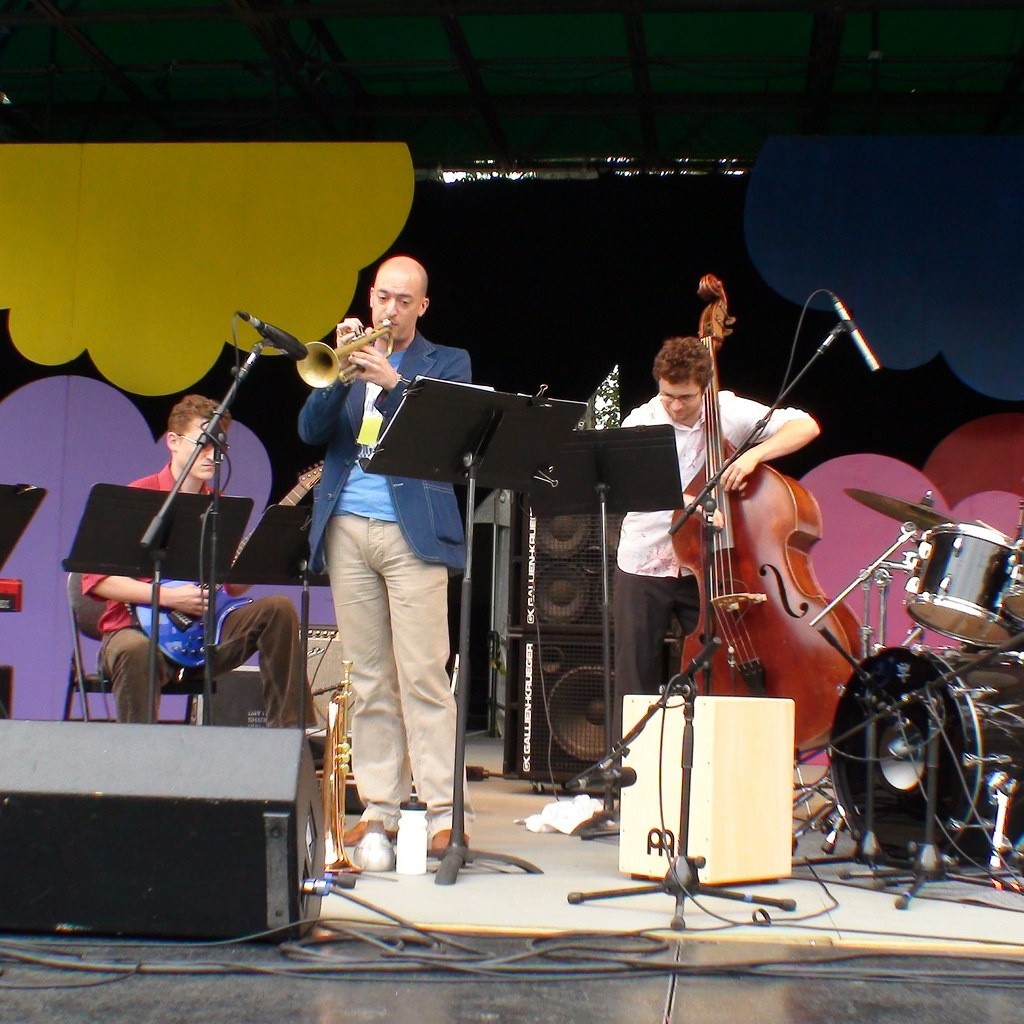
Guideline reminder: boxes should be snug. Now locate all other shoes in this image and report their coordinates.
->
[426,830,470,859]
[344,821,397,847]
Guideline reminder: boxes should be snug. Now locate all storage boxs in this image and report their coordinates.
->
[619,694,797,887]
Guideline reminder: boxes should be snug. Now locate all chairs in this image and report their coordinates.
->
[60,572,218,723]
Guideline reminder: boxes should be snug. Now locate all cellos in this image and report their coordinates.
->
[671,271,863,830]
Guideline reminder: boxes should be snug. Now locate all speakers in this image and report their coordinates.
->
[498,491,626,785]
[0,624,356,945]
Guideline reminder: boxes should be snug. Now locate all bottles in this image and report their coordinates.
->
[396,793,427,875]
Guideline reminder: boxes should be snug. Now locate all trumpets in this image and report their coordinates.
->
[322,660,363,873]
[297,318,394,389]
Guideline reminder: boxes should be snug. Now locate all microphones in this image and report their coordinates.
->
[887,716,924,761]
[829,291,880,370]
[236,311,309,361]
[565,767,638,788]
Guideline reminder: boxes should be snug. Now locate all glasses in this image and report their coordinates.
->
[659,387,703,402]
[179,436,230,454]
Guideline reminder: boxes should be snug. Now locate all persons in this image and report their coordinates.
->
[81,395,317,729]
[298,256,476,847]
[596,337,819,823]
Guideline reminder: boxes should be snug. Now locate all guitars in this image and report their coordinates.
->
[125,459,325,668]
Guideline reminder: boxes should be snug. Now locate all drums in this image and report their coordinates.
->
[904,520,1023,649]
[828,648,1024,868]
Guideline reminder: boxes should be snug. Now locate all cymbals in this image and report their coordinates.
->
[844,488,957,530]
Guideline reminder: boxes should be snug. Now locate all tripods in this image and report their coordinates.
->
[355,375,588,888]
[567,638,797,933]
[792,631,1024,910]
